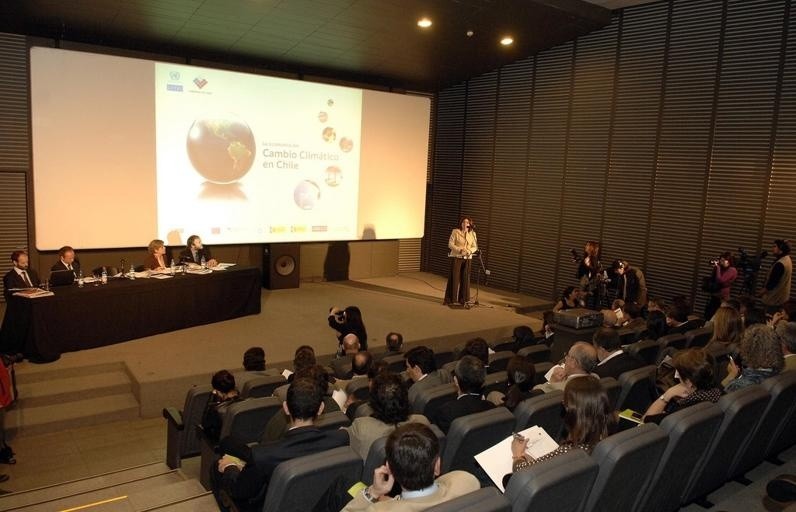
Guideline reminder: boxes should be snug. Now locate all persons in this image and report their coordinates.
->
[51,246,80,281]
[199,239,796,512]
[3,251,39,301]
[179,235,217,269]
[144,240,171,270]
[442,216,478,305]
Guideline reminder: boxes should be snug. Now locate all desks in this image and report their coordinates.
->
[0,263,264,365]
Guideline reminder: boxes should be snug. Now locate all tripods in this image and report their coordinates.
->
[466,229,493,310]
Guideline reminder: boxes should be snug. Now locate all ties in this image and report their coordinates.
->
[23,271,31,287]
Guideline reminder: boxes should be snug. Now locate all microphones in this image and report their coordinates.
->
[467,226,472,228]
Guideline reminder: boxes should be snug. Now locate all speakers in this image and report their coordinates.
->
[263,243,301,290]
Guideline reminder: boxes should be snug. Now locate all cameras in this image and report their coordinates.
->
[330,308,344,321]
[3,352,23,367]
[710,259,720,265]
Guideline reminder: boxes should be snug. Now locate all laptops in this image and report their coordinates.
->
[38,270,73,287]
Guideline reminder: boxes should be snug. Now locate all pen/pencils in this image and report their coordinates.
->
[511,432,529,449]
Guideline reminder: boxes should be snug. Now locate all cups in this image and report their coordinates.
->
[93,273,99,286]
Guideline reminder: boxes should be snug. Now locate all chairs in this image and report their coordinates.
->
[161,318,796,510]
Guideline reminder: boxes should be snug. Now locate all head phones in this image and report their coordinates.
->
[617,260,625,269]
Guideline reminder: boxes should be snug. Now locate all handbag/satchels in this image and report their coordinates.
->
[650,368,680,401]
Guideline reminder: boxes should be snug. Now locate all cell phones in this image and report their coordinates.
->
[631,412,642,420]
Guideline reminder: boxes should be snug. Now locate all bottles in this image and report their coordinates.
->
[120,264,125,278]
[101,266,107,285]
[201,255,206,270]
[44,279,49,291]
[170,258,175,275]
[78,270,84,287]
[129,263,135,281]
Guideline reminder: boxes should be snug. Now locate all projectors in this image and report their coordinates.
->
[560,307,604,329]
